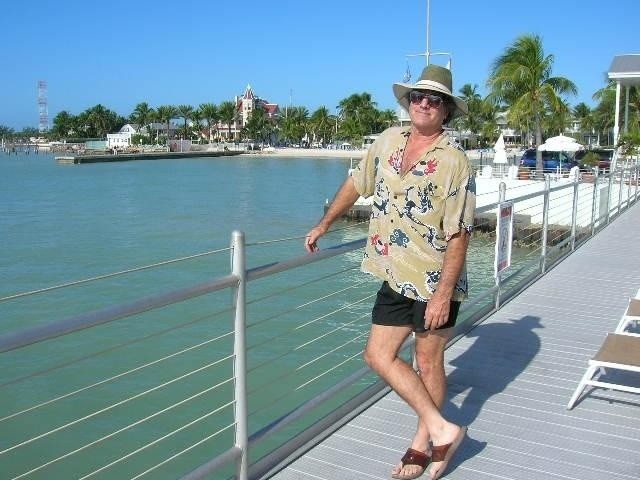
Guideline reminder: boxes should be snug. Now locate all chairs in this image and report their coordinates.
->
[474,164,583,182]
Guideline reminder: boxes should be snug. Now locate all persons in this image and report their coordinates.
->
[303,64,479,480]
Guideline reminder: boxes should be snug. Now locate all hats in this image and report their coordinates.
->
[392,64,469,122]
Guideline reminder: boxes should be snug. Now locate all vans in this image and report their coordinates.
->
[521,150,593,175]
[574,148,616,168]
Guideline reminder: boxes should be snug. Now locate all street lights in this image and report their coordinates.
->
[180,134,184,152]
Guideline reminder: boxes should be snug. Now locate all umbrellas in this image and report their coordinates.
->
[492,133,508,175]
[537,132,585,174]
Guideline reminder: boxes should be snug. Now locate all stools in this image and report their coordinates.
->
[635,286,640,298]
[614,298,640,332]
[569,333,640,411]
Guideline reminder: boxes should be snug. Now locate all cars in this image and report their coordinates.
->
[258,141,324,148]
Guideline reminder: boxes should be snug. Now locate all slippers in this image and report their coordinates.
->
[429,424,468,480]
[392,446,432,480]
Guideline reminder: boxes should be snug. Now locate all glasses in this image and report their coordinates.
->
[410,91,445,109]
